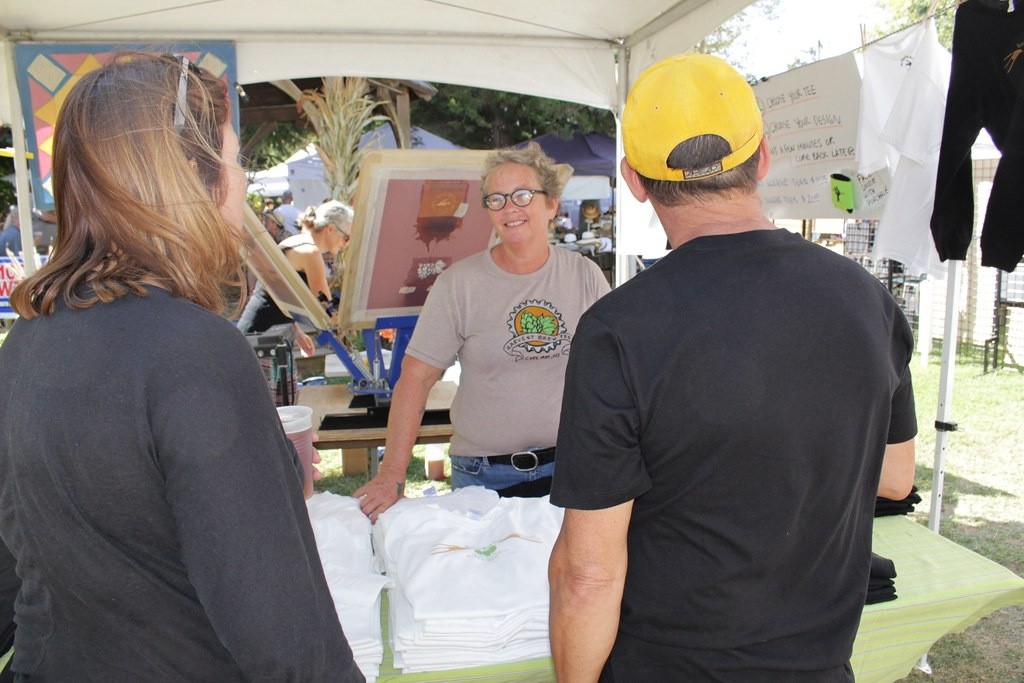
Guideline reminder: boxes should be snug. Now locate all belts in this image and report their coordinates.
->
[478,447,556,471]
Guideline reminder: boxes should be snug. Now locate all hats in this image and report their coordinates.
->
[266,212,284,226]
[622,55,764,182]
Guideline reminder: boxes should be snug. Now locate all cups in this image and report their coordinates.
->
[275,405,314,500]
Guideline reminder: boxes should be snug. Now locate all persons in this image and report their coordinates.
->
[548,54,917,683]
[351,147,614,522]
[0,54,353,683]
[558,211,572,230]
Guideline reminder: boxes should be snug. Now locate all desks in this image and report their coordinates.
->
[292,380,457,475]
[376,509,1024,683]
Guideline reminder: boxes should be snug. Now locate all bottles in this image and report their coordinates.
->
[426,443,444,480]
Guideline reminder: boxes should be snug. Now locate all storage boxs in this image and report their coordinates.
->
[424,443,444,481]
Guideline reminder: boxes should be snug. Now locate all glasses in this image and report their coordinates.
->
[336,226,351,242]
[481,189,548,211]
[161,53,203,136]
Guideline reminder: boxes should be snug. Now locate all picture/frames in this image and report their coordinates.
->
[14,38,241,211]
[238,202,334,331]
[337,147,518,332]
